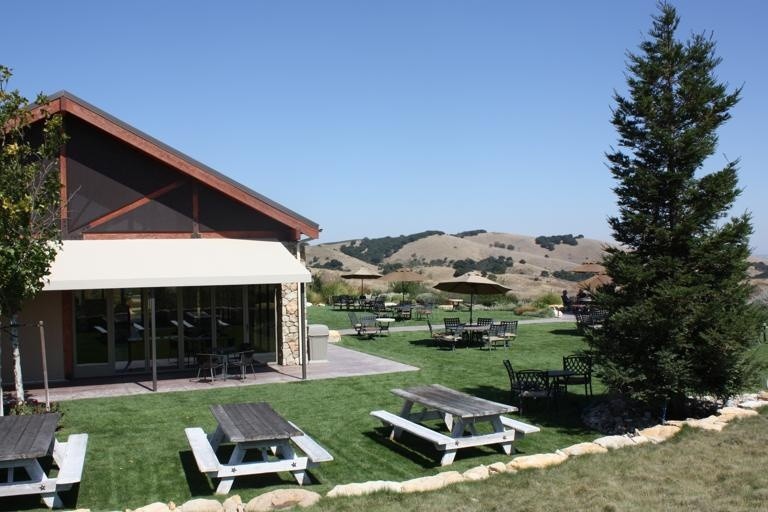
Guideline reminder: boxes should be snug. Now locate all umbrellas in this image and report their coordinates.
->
[340,266,384,297]
[433,270,512,345]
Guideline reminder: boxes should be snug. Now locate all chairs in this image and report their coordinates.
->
[194,351,228,384]
[226,347,256,382]
[326,292,520,352]
[577,308,610,335]
[563,352,594,399]
[503,356,550,413]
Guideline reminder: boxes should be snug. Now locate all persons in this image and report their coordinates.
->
[561,289,574,307]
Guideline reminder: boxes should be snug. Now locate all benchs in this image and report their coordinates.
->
[368,382,541,465]
[0,408,92,510]
[178,395,333,500]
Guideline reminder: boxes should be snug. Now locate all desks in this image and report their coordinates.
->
[535,367,577,403]
